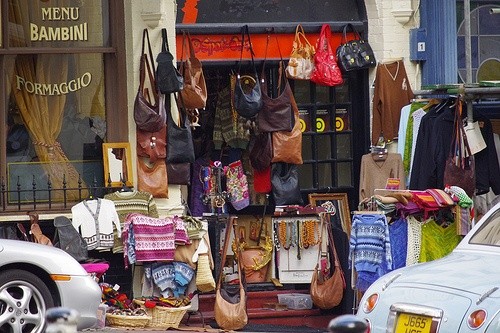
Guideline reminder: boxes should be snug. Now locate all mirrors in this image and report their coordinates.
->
[103,142,134,188]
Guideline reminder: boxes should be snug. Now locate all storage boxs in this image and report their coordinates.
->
[277,293,312,311]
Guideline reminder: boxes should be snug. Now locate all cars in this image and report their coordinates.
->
[0,238,107,333]
[351,198,499,333]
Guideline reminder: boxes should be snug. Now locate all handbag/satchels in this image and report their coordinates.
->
[249,133,273,171]
[310,23,344,87]
[213,282,249,331]
[167,160,191,186]
[234,24,262,118]
[336,21,376,73]
[311,213,344,309]
[282,23,315,80]
[270,164,306,206]
[269,117,303,164]
[134,53,165,132]
[178,30,208,110]
[137,124,167,158]
[167,118,197,162]
[137,157,168,198]
[257,87,292,132]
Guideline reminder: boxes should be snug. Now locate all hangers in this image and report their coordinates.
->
[86,183,97,201]
[361,139,402,160]
[352,201,450,226]
[120,183,132,192]
[399,94,488,123]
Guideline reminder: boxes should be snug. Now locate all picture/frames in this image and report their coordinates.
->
[310,192,352,242]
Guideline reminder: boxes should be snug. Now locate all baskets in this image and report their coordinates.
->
[132,297,192,331]
[104,312,151,329]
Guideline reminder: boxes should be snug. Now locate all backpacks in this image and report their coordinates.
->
[155,28,183,94]
